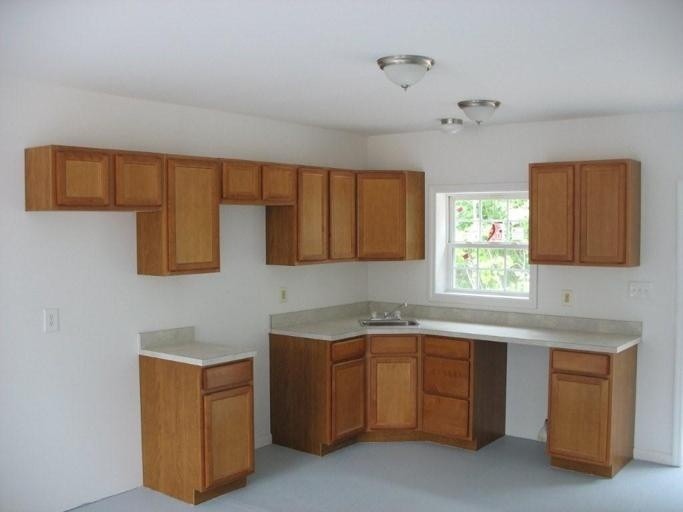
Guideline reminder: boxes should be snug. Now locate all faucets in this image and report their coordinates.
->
[384,302,408,320]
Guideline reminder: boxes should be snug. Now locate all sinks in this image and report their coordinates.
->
[359,317,420,328]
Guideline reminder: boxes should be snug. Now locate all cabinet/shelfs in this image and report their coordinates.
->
[528,158,642,269]
[547,342,639,479]
[137,353,254,505]
[269,333,507,457]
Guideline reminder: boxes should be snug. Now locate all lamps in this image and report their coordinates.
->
[439,117,463,134]
[456,99,501,125]
[374,54,435,94]
